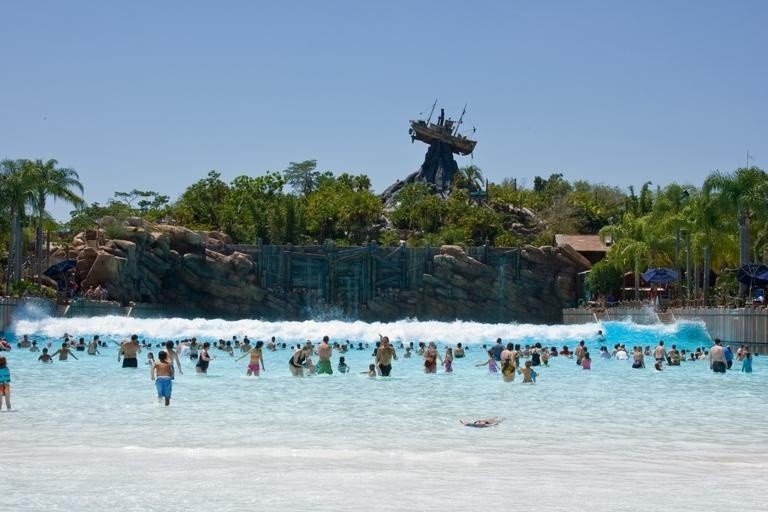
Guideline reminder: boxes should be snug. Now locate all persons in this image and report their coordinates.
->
[37,348,52,363]
[403,347,412,358]
[541,348,550,365]
[210,342,217,349]
[454,342,466,358]
[569,350,574,356]
[150,350,174,406]
[655,341,668,363]
[218,340,225,349]
[424,342,443,374]
[239,339,251,352]
[514,344,524,369]
[680,349,686,360]
[720,344,733,369]
[652,362,662,373]
[29,340,39,353]
[631,346,646,368]
[575,339,585,365]
[144,352,154,367]
[197,345,203,361]
[459,416,501,429]
[119,335,141,367]
[181,339,191,348]
[531,342,543,365]
[667,344,676,358]
[289,346,312,378]
[235,340,265,377]
[579,346,588,364]
[0,338,9,352]
[738,345,749,362]
[631,346,637,353]
[366,363,375,377]
[68,334,75,339]
[97,340,102,346]
[303,339,314,352]
[86,335,99,355]
[726,345,733,359]
[374,332,382,352]
[142,338,161,349]
[102,341,107,347]
[612,343,628,360]
[337,356,351,375]
[0,356,11,410]
[85,286,94,298]
[190,337,198,361]
[46,341,52,347]
[135,340,139,346]
[671,350,680,364]
[599,345,611,360]
[333,339,370,354]
[95,285,102,298]
[518,359,539,384]
[162,340,184,375]
[59,333,68,338]
[22,335,31,348]
[703,351,708,359]
[740,352,752,373]
[2,339,12,348]
[316,335,333,376]
[416,342,425,356]
[581,352,592,369]
[551,347,558,357]
[695,347,701,358]
[398,342,404,348]
[444,348,454,372]
[644,345,651,355]
[688,353,695,361]
[522,344,532,357]
[196,342,213,374]
[70,339,75,346]
[266,335,302,350]
[476,350,500,376]
[501,343,517,383]
[374,337,399,376]
[51,342,79,362]
[737,345,744,358]
[710,338,728,373]
[224,342,233,353]
[409,341,413,351]
[175,340,181,353]
[561,345,570,356]
[593,329,606,342]
[77,337,86,351]
[489,337,505,361]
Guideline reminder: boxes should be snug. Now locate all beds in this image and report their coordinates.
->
[733,264,768,299]
[639,266,677,299]
[43,259,75,290]
